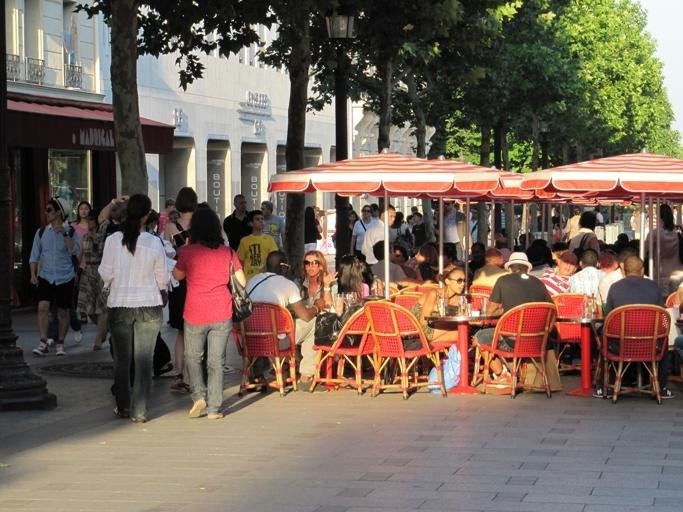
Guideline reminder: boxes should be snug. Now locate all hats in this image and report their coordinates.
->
[504,252,533,274]
[556,251,578,265]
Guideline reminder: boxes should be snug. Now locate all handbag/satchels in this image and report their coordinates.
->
[229,273,253,322]
[574,247,584,256]
[428,344,461,395]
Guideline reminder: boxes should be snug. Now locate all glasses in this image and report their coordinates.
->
[448,277,466,284]
[303,259,321,269]
[280,262,291,271]
[44,207,54,212]
[444,201,452,205]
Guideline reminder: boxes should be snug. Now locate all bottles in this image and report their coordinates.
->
[590,293,598,318]
[583,295,589,318]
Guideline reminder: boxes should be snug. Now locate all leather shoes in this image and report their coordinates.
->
[94,327,109,351]
[154,364,173,376]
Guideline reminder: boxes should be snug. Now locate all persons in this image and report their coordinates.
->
[591,257,674,400]
[28,186,285,393]
[296,203,683,358]
[98,194,168,423]
[172,208,247,421]
[245,251,329,393]
[473,253,554,389]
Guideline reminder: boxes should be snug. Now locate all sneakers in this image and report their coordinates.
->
[563,353,572,365]
[113,406,145,423]
[659,388,677,399]
[169,373,224,419]
[593,385,613,399]
[74,329,82,343]
[32,338,66,356]
[223,365,241,375]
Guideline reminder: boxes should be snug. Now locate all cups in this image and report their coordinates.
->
[438,300,445,316]
[459,296,467,316]
[481,298,489,315]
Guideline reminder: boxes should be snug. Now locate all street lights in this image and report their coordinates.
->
[324,3,359,273]
[538,125,552,231]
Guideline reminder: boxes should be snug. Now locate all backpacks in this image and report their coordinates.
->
[315,312,339,345]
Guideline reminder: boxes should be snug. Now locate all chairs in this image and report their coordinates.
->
[364,300,447,399]
[470,285,502,326]
[551,293,604,371]
[375,281,442,313]
[240,301,295,396]
[666,292,677,308]
[479,302,556,399]
[603,303,671,404]
[307,299,388,393]
